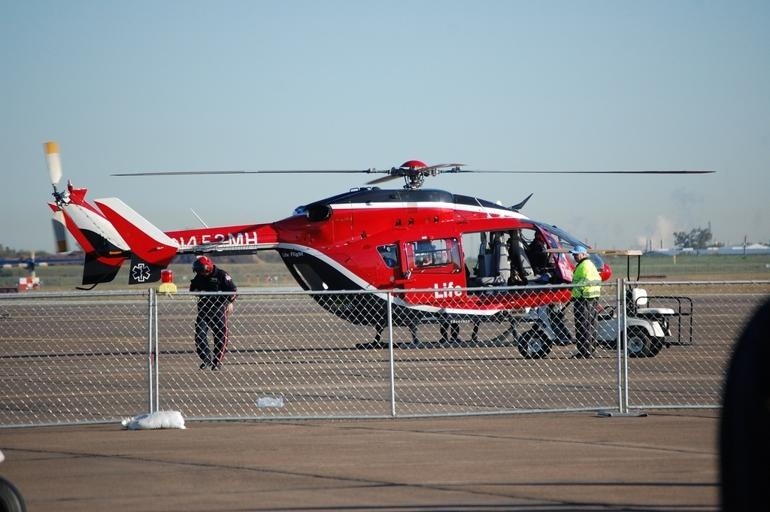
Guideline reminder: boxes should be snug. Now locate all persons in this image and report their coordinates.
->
[568,243,603,360]
[437,322,462,343]
[188,255,238,373]
[526,227,563,284]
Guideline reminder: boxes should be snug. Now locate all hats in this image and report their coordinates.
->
[568,246,587,255]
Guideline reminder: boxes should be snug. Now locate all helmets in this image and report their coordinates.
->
[192,255,214,277]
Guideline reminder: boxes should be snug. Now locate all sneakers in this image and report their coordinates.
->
[200,360,211,370]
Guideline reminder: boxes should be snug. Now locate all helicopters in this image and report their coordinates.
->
[45,140,718,350]
